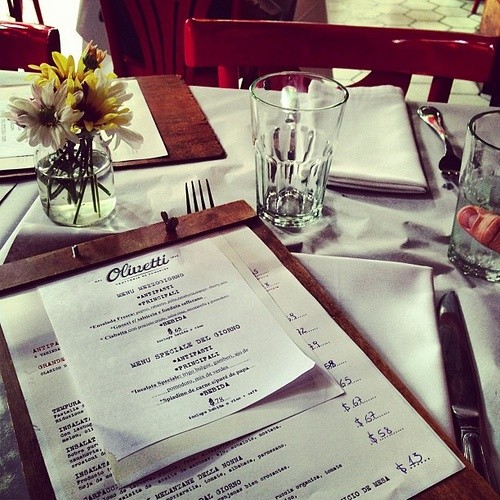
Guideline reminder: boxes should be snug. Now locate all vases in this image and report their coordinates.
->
[33,128,116,228]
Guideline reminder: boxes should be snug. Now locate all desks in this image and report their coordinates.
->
[0,69,500,500]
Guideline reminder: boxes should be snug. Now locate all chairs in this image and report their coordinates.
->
[183,18,496,104]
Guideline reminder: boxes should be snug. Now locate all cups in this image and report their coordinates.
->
[248,71,349,229]
[447,110,500,283]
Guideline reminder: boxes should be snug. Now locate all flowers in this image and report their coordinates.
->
[0,38,144,152]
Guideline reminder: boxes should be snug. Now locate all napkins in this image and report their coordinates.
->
[295,80,431,194]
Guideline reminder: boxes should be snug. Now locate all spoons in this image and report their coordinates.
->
[273,85,298,162]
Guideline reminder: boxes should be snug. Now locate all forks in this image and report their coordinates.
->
[185,179,215,215]
[417,105,464,176]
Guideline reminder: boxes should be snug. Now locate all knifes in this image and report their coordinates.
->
[435,290,492,487]
